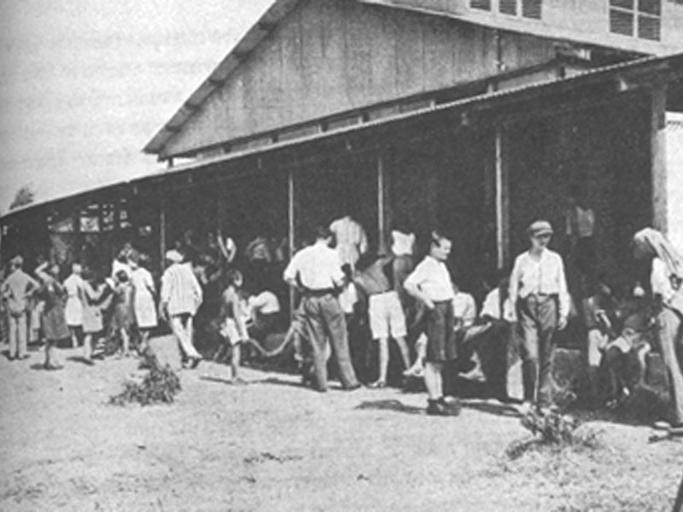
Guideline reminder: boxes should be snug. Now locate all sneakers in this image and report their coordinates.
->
[427,401,459,415]
[315,366,422,392]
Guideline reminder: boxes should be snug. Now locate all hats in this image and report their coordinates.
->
[166,250,182,261]
[528,222,553,236]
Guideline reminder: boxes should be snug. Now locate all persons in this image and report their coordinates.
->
[577,227,680,443]
[201,212,570,415]
[0,242,203,370]
